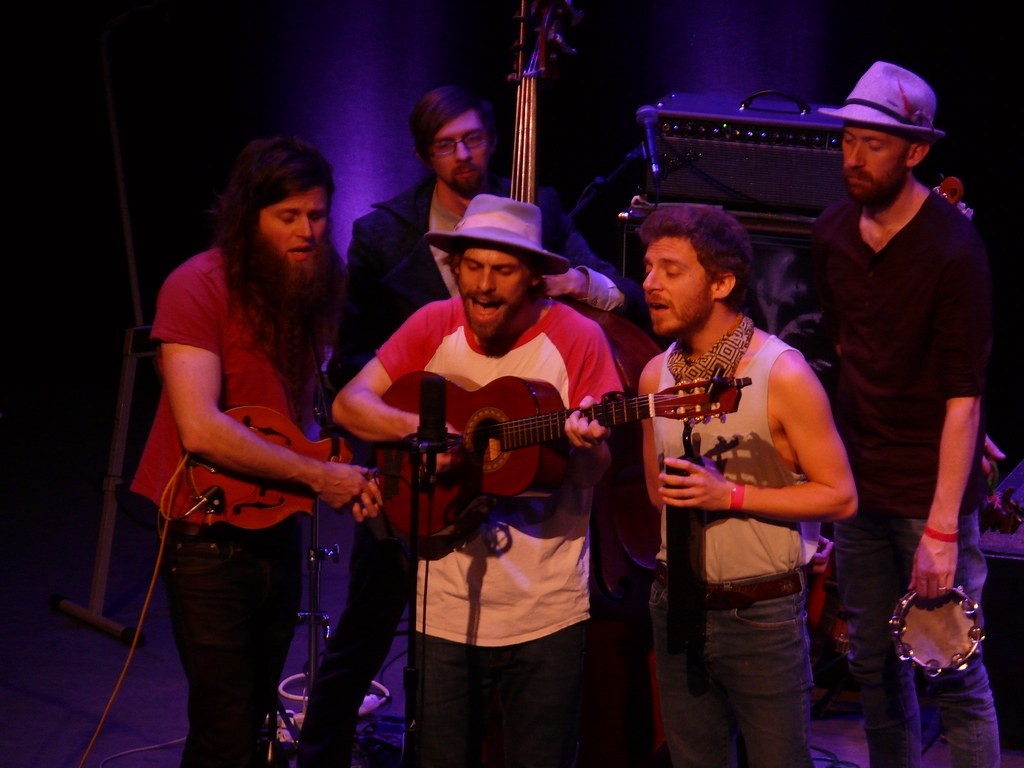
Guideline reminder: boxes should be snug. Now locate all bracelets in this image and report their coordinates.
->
[730,484,745,511]
[925,526,959,542]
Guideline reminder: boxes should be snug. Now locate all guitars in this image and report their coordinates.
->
[357,371,752,540]
[164,398,387,553]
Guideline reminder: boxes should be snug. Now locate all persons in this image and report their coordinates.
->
[810,61,1001,768]
[130,136,384,768]
[296,86,651,768]
[638,204,859,768]
[331,192,624,768]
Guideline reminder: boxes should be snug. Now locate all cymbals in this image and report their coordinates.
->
[886,615,908,636]
[923,659,942,677]
[897,641,913,661]
[961,598,980,615]
[968,625,987,642]
[950,652,968,672]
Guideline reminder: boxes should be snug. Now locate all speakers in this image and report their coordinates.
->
[587,221,844,405]
[974,459,1024,752]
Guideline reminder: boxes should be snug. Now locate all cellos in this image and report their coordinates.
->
[498,0,590,247]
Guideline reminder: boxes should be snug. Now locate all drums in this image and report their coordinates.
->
[893,588,980,668]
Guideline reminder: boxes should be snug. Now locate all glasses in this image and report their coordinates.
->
[426,130,490,156]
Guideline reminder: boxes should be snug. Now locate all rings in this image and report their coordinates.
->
[939,588,946,590]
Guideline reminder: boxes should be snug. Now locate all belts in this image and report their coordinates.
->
[655,562,808,602]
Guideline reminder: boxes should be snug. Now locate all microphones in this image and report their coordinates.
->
[416,377,446,491]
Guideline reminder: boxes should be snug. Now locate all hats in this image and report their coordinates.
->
[424,193,570,275]
[816,61,947,140]
